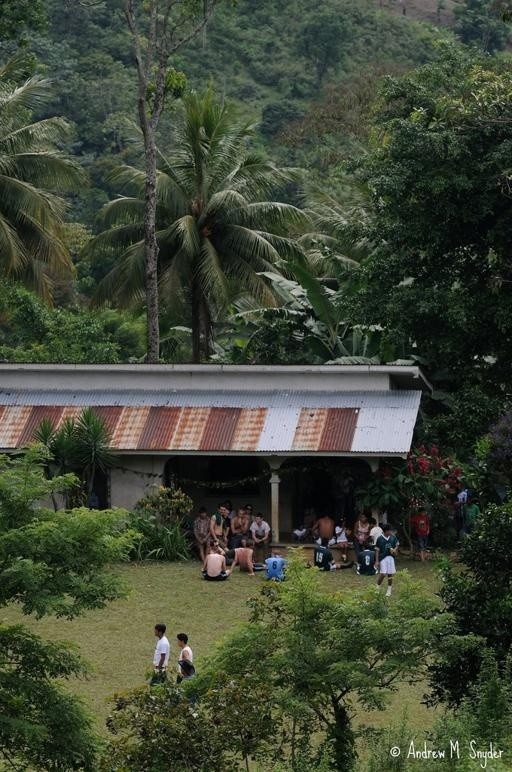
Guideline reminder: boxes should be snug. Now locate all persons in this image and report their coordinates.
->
[462,495,480,537]
[374,523,400,596]
[150,624,170,686]
[411,508,430,561]
[292,506,384,576]
[179,659,195,677]
[264,550,287,582]
[177,633,193,684]
[192,501,271,581]
